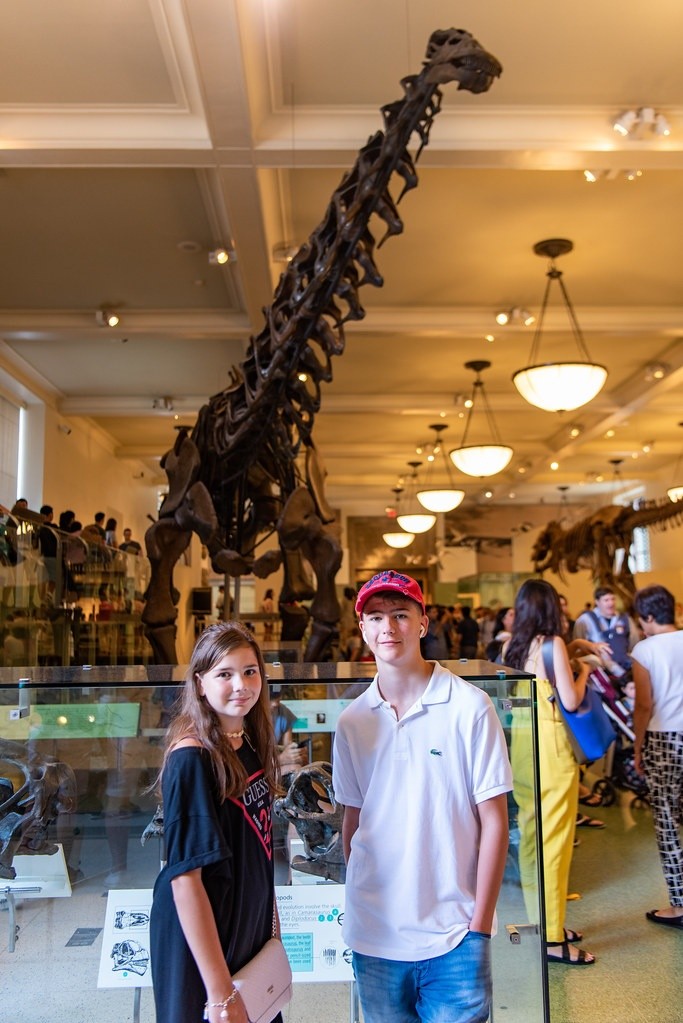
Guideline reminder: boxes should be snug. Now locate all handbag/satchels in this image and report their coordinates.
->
[541,635,618,765]
[203,937,293,1023]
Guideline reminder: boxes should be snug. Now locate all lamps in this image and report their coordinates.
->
[209,245,232,267]
[666,422,683,503]
[383,488,416,549]
[94,309,120,327]
[614,104,671,135]
[394,461,438,537]
[273,243,299,264]
[646,360,668,383]
[581,169,645,182]
[448,359,515,478]
[494,238,609,413]
[414,423,465,513]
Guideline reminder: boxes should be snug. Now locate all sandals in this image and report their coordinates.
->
[563,928,582,942]
[547,941,596,964]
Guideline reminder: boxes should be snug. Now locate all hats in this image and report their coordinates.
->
[354,571,426,616]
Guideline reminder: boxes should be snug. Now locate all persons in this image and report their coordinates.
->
[216,584,234,622]
[504,578,612,966]
[261,588,274,641]
[334,569,517,1023]
[0,606,99,668]
[149,619,286,1023]
[5,498,144,599]
[630,585,683,926]
[323,586,647,846]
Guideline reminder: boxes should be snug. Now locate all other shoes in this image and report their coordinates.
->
[646,910,683,930]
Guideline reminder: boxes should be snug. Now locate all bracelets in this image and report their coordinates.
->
[203,984,237,1020]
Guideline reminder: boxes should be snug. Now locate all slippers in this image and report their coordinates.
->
[576,813,606,829]
[579,791,603,807]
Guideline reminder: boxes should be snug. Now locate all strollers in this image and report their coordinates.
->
[572,666,654,810]
